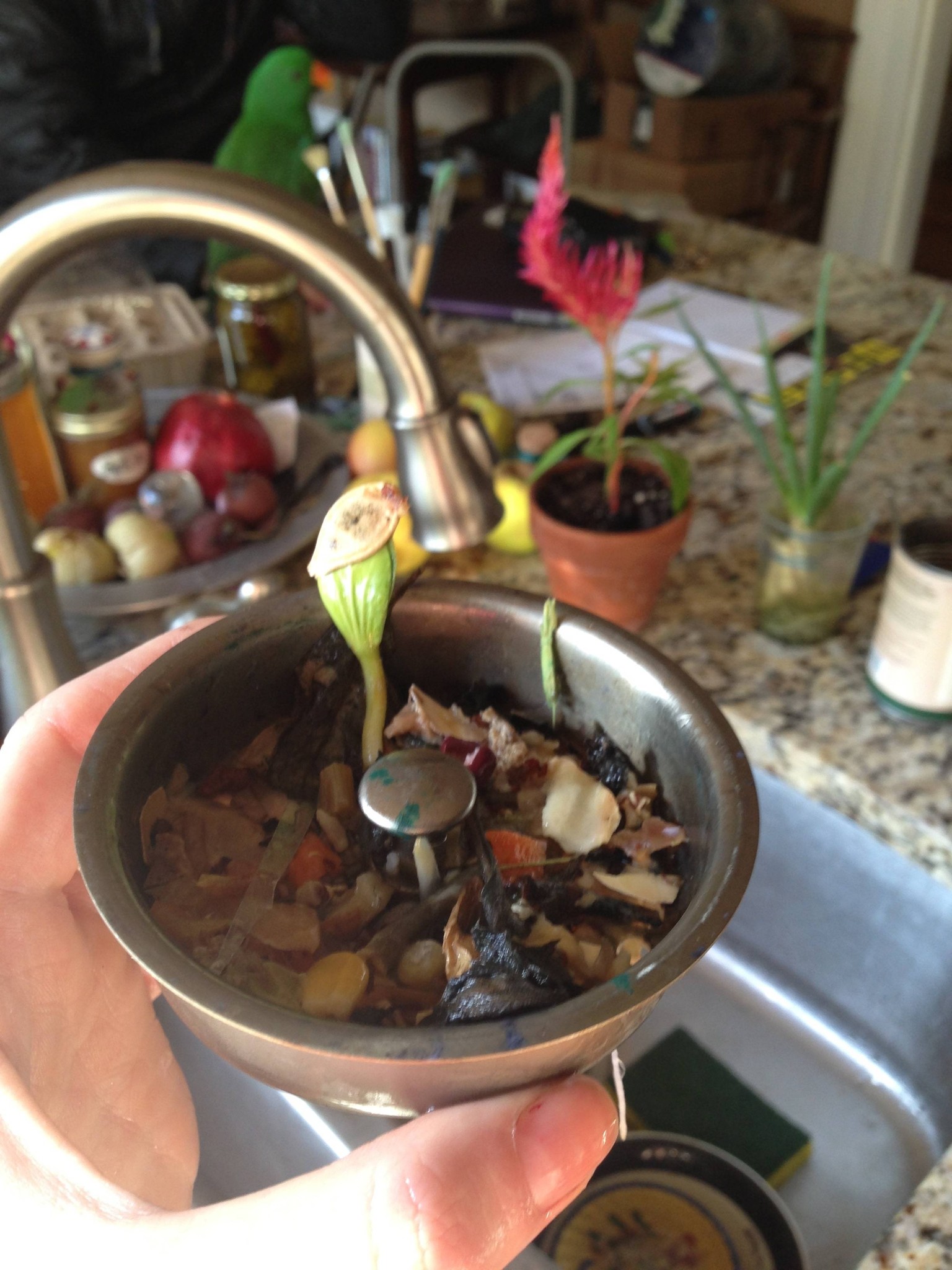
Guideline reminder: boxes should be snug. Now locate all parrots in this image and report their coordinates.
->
[204,46,340,290]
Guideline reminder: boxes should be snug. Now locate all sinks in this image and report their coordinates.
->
[104,755,952,1270]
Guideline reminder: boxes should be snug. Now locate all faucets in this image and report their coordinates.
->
[0,159,504,715]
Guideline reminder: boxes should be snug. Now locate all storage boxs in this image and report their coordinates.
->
[567,126,833,221]
[601,80,809,165]
[18,280,214,395]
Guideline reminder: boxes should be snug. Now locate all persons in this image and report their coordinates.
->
[0,614,618,1270]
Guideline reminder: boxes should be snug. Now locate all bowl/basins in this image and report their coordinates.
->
[77,579,761,1123]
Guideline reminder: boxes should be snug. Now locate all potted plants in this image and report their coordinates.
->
[676,252,947,649]
[508,117,697,654]
